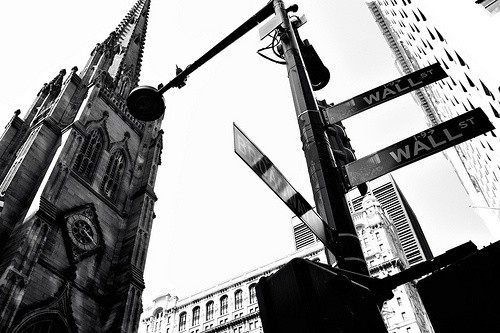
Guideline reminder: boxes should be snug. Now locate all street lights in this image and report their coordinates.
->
[126,0,388,333]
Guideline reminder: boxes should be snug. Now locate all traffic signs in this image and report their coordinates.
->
[343,108,496,186]
[233,121,343,260]
[325,63,449,123]
